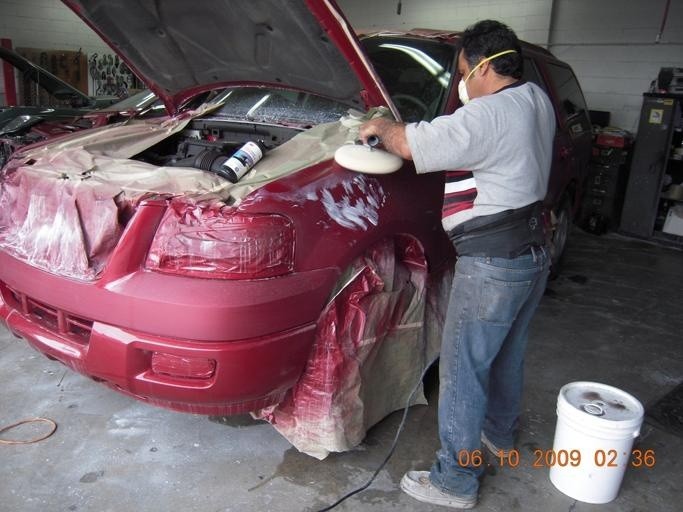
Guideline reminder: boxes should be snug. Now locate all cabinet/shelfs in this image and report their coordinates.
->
[615,92,683,247]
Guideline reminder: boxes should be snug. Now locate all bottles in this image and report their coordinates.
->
[220,139,265,181]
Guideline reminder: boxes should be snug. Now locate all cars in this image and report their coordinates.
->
[0,42,172,166]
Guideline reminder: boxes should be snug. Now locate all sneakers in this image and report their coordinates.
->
[401,470,477,509]
[481,432,516,458]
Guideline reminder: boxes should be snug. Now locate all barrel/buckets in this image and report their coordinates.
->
[549,379,645,504]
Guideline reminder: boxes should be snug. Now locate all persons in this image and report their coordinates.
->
[356,19,560,509]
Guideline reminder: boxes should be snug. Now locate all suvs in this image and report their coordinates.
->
[0,0,600,430]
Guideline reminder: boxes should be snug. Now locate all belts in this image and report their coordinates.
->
[520,247,545,257]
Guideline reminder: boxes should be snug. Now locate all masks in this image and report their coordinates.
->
[458,49,516,104]
